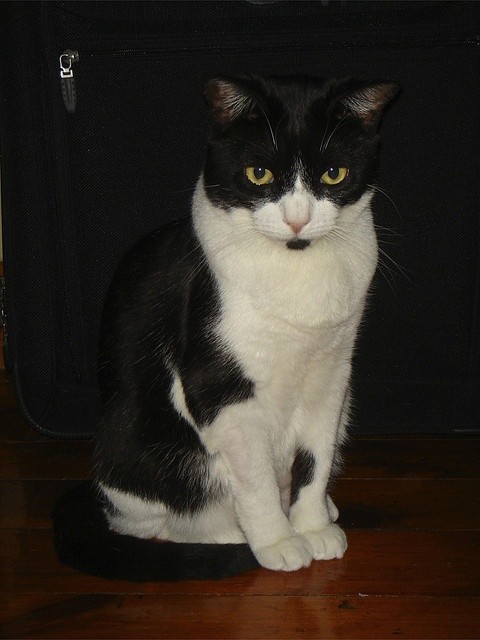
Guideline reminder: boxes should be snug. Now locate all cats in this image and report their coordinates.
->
[51,70,402,580]
